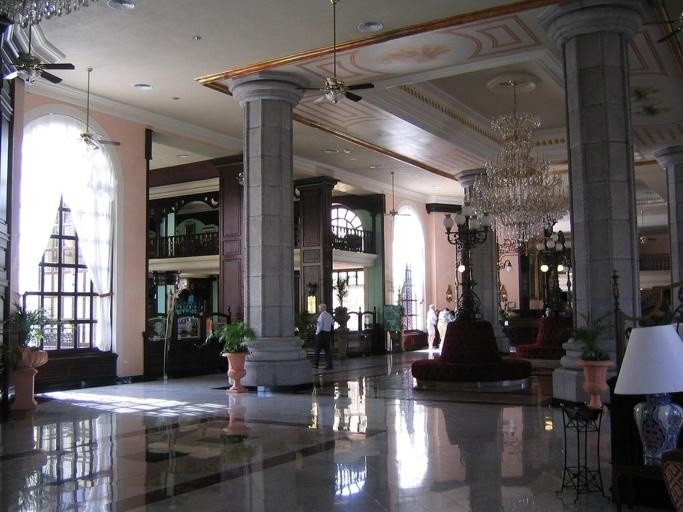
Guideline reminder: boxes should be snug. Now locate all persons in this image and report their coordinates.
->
[311,304,334,370]
[437,308,457,353]
[426,304,437,351]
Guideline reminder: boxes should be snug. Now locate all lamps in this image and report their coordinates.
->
[470,69,568,246]
[443,204,492,320]
[536,233,571,313]
[612,325,682,464]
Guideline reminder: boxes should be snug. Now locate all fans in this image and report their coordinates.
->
[76,68,120,151]
[3,21,74,85]
[294,0,374,105]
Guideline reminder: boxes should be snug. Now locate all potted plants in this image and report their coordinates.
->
[0,301,78,410]
[566,325,617,411]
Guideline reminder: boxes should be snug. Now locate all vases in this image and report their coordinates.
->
[211,320,257,393]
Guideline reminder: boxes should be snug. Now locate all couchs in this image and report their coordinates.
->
[411,313,567,393]
